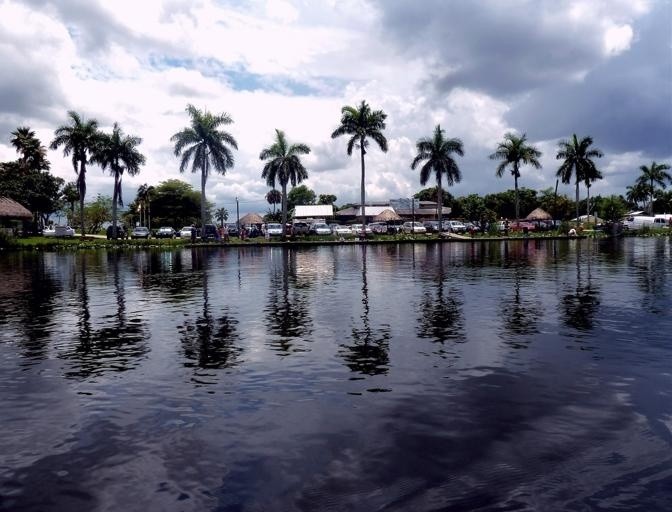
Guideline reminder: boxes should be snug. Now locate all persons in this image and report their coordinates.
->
[214,223,229,244]
[191,224,197,241]
[240,225,246,239]
[359,229,366,240]
[377,226,387,234]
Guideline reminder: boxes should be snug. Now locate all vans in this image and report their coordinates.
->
[204,224,218,238]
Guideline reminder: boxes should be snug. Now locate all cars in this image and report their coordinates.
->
[593,221,606,230]
[131,226,152,240]
[155,226,177,239]
[180,226,197,237]
[13,225,75,238]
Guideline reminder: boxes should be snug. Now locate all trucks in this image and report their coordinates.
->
[623,212,672,232]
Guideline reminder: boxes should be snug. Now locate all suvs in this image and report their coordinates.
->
[106,224,129,240]
